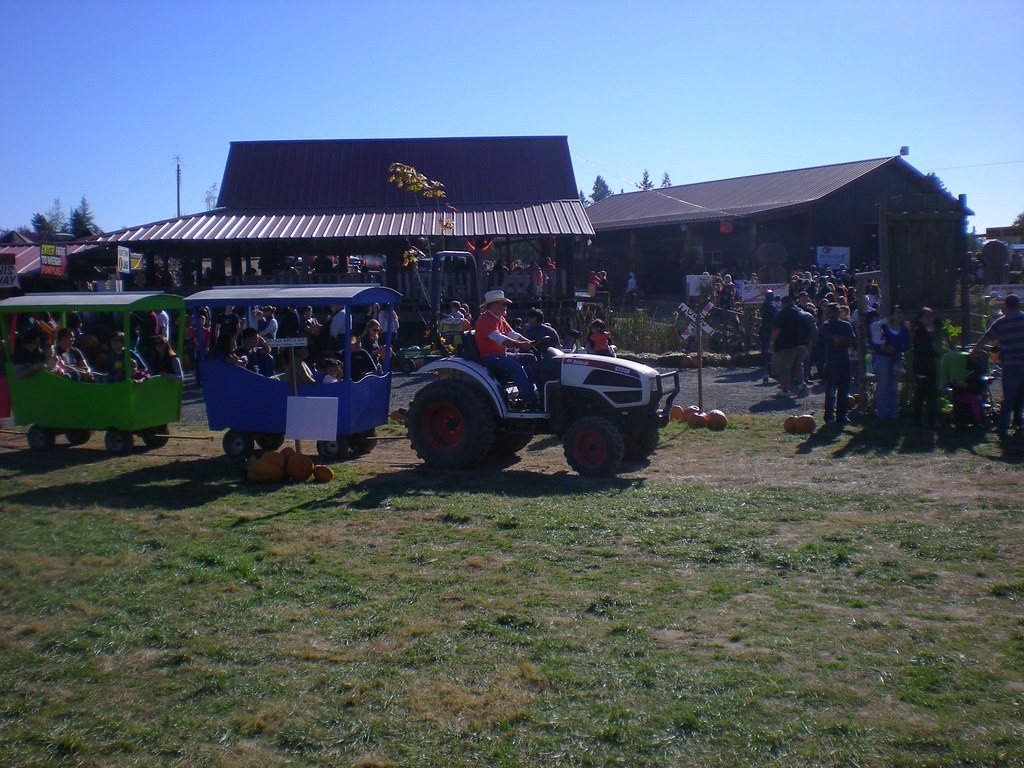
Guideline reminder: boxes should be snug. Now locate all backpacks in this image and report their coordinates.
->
[785,308,810,341]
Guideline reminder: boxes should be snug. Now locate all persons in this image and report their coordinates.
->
[442,290,618,411]
[589,270,609,291]
[910,306,941,431]
[14,252,401,384]
[972,291,1024,438]
[624,271,641,311]
[443,255,556,287]
[702,263,910,427]
[968,251,1024,285]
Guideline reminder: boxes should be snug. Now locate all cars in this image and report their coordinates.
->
[346,254,387,275]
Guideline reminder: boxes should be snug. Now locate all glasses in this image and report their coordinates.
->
[110,339,120,343]
[372,327,380,332]
[498,302,507,307]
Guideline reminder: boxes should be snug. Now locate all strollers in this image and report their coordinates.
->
[942,350,999,431]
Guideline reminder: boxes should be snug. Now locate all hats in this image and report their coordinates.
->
[479,290,513,308]
[799,291,809,297]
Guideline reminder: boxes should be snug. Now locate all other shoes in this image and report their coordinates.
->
[526,403,540,409]
